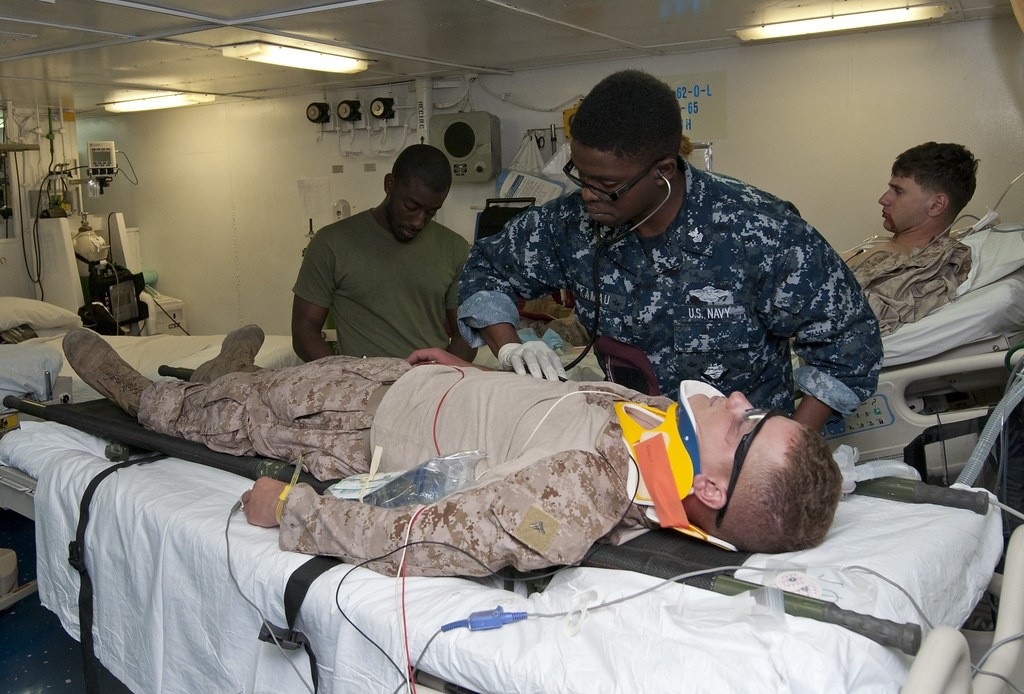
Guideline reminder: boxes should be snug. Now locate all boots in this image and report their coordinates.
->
[189,323,264,384]
[62,328,155,420]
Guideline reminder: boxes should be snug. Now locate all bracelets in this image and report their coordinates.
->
[275,458,303,525]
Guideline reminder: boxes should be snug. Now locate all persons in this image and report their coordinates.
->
[836,142,982,337]
[456,70,885,436]
[292,145,479,362]
[63,322,845,581]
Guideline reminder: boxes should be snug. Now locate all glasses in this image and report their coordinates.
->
[563,157,669,202]
[715,405,789,529]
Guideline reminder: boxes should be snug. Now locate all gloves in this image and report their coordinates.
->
[499,340,568,382]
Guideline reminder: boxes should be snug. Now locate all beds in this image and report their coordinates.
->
[1,413,1024,694]
[1,330,338,405]
[473,266,1024,467]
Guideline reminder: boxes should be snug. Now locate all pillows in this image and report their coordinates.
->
[0,296,83,332]
[956,223,1024,298]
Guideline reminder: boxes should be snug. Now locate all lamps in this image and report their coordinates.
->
[734,5,948,41]
[337,100,362,122]
[222,42,368,74]
[104,93,217,113]
[305,102,331,123]
[369,96,394,120]
